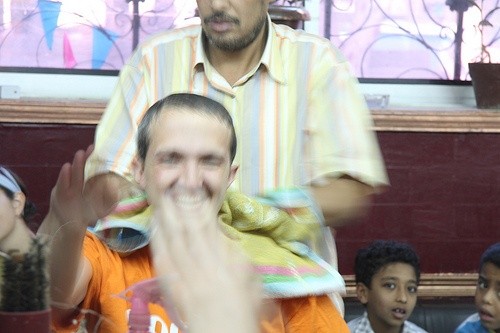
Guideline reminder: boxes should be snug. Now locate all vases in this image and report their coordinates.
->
[469,62,500,109]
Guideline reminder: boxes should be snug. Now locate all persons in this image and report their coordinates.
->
[84,0,391,314]
[47,93,353,332]
[146,186,266,332]
[456,244,500,332]
[346,240,428,333]
[0,164,52,308]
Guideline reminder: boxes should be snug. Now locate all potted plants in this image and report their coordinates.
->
[0,236,53,333]
